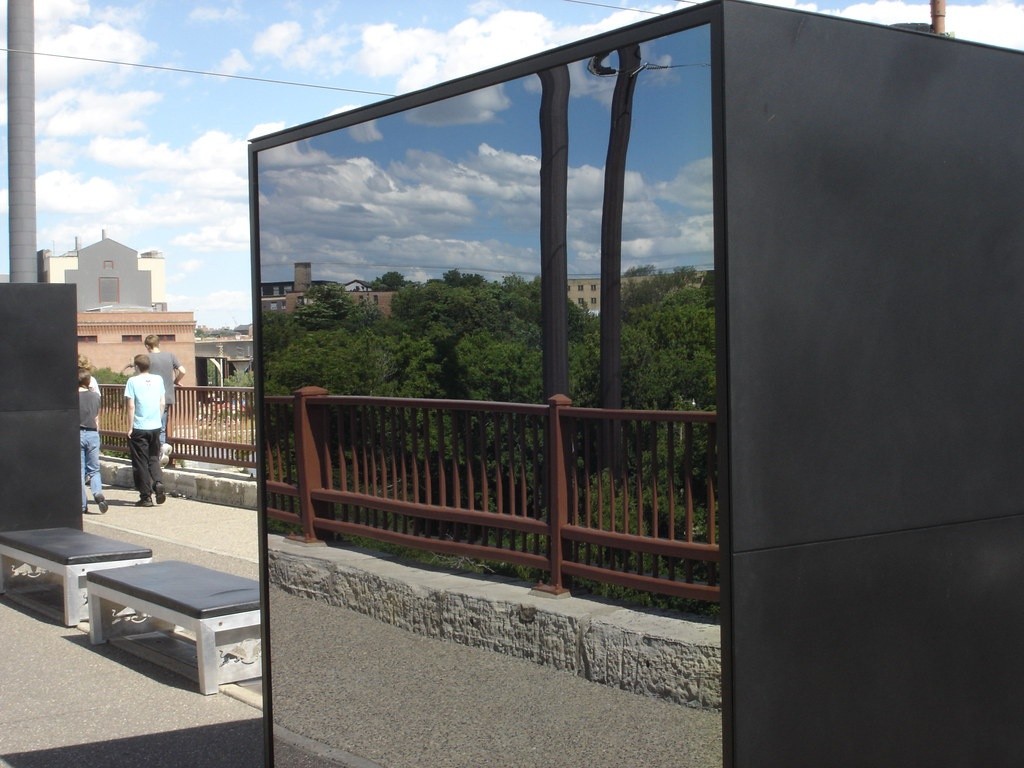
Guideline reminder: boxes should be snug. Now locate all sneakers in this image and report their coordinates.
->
[159,444,173,466]
[155,481,165,504]
[136,496,153,507]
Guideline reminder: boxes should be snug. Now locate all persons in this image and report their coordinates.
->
[142,335,187,468]
[124,353,167,506]
[78,367,108,514]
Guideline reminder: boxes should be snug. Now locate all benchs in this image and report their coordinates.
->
[0,527,153,626]
[89,560,260,695]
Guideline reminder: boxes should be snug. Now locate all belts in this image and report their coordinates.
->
[81,427,97,431]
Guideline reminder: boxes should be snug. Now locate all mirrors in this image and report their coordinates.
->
[246,0,736,768]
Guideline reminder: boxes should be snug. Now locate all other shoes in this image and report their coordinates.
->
[96,494,108,513]
